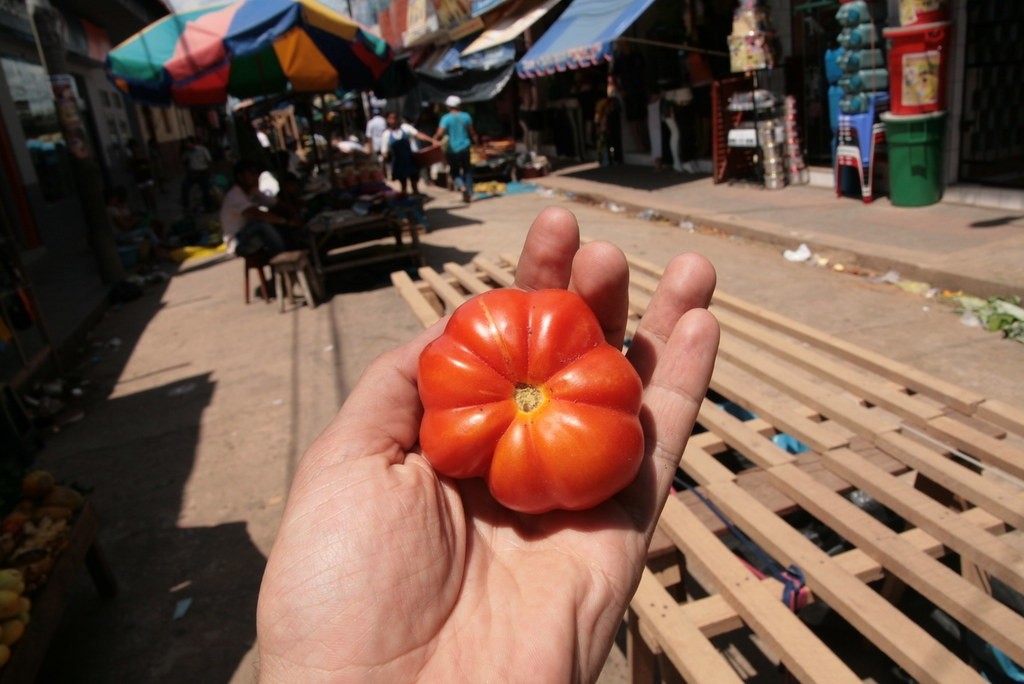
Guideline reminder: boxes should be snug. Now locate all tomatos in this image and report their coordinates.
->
[418,287,645,516]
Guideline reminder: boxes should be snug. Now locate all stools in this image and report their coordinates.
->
[242,251,322,313]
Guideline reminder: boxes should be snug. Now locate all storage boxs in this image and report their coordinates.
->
[414,144,442,168]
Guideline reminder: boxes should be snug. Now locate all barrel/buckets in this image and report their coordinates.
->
[879,0,948,208]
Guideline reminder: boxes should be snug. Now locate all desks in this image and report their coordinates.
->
[0,501,117,684]
[308,203,427,298]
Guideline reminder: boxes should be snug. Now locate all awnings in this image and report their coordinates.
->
[460,1,656,80]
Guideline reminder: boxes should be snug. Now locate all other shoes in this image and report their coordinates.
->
[470,195,476,201]
[460,185,469,201]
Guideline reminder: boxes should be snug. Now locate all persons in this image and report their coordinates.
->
[256,207,721,684]
[32,75,622,290]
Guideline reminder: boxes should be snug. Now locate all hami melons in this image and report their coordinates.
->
[15,469,83,524]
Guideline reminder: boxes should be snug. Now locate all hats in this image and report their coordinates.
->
[445,95,462,108]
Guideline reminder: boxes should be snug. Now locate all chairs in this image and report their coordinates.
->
[832,92,890,203]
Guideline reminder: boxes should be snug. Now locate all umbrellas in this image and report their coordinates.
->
[107,1,394,111]
